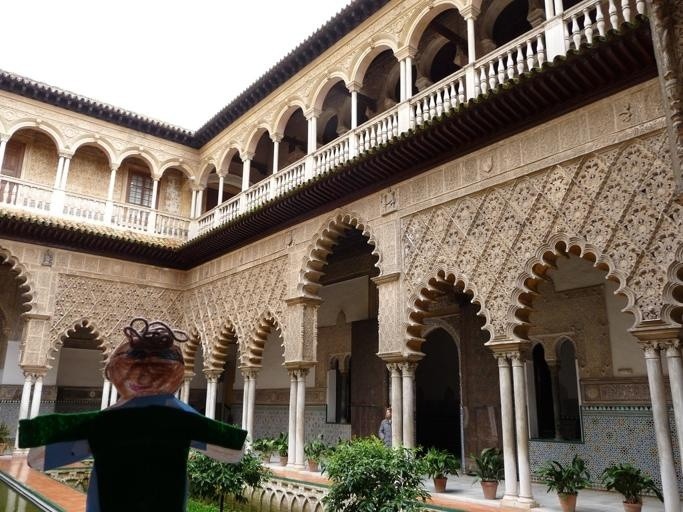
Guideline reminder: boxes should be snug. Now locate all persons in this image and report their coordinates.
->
[377,406,391,448]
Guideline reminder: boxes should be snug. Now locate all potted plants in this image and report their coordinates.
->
[531,454,595,512]
[250,437,275,463]
[0,421,12,456]
[304,434,326,472]
[272,432,289,465]
[422,445,462,492]
[466,447,504,500]
[598,462,664,512]
